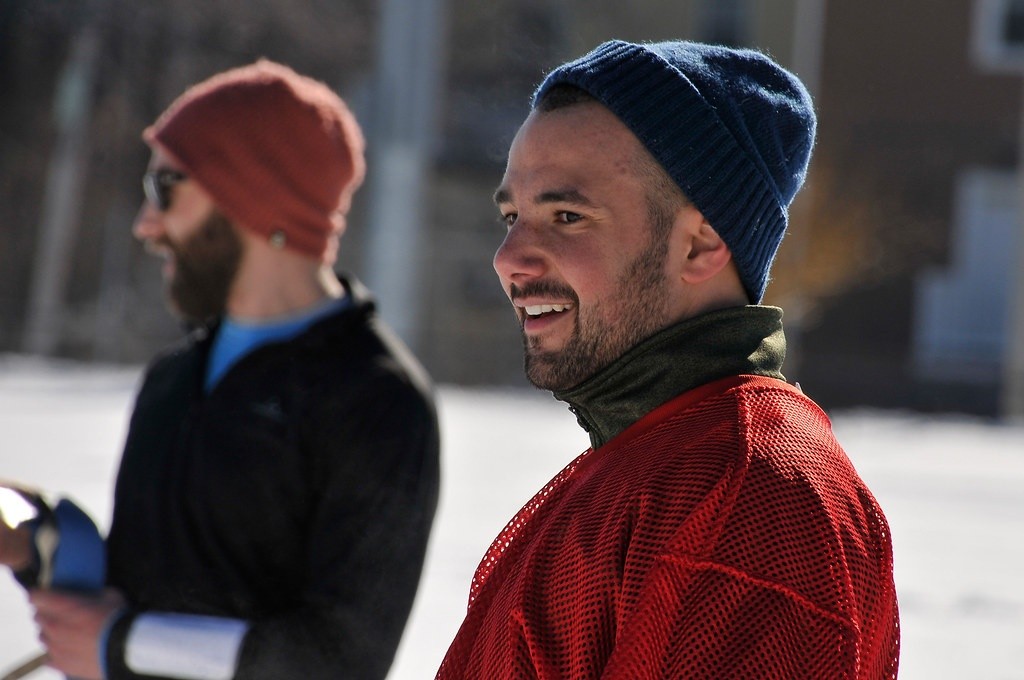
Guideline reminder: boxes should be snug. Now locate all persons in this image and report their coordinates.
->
[0,58,443,679]
[435,39,899,679]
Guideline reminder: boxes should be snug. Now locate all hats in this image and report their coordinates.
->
[143,58,366,267]
[532,39,817,305]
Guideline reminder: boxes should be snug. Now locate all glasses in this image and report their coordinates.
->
[142,169,192,210]
